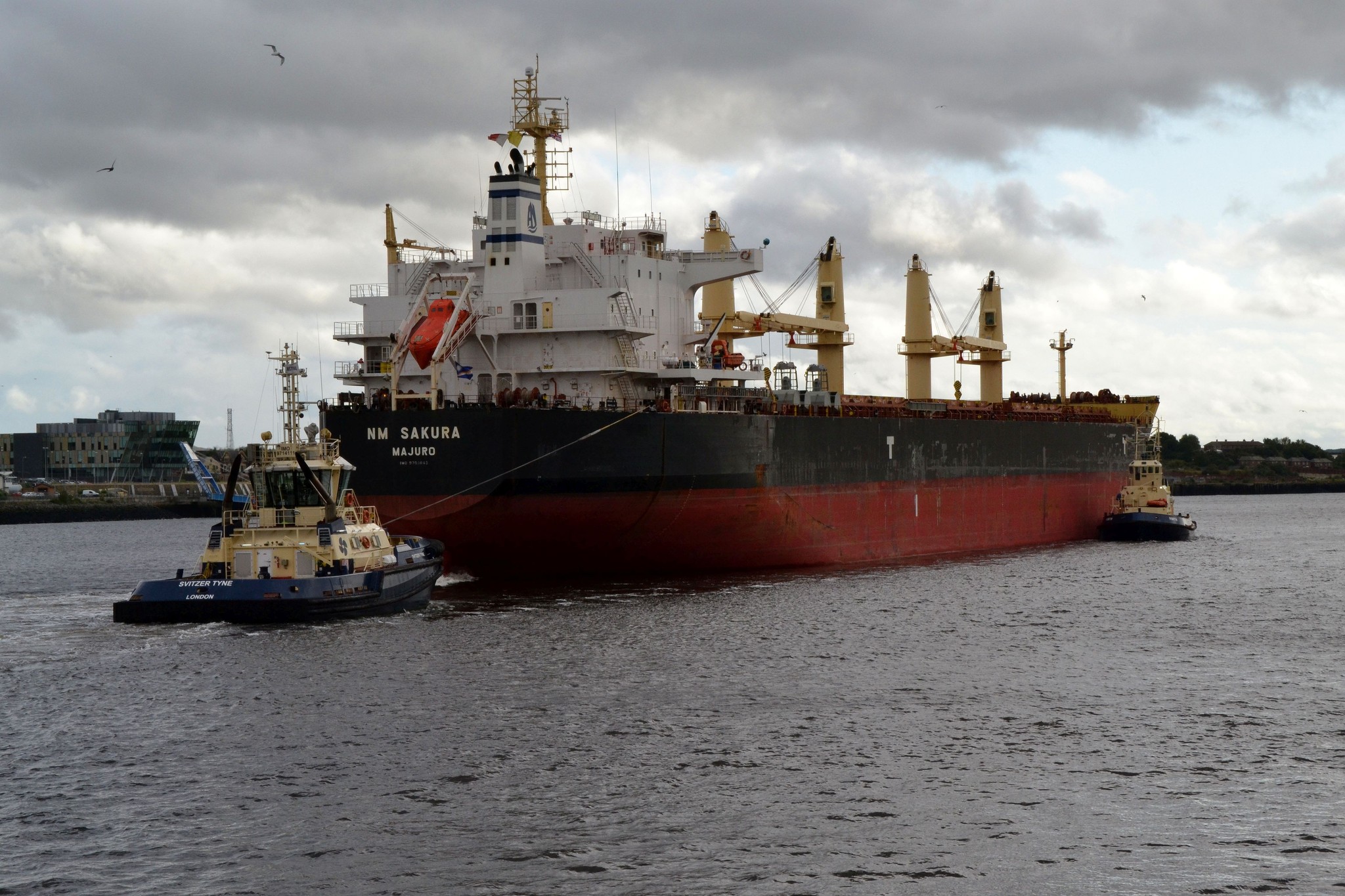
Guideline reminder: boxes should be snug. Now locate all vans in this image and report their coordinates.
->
[82,490,100,497]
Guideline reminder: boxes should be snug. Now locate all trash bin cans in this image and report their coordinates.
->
[186,488,189,494]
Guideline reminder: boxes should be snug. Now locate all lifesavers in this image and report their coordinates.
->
[662,401,669,410]
[346,493,353,506]
[362,536,370,548]
[363,510,370,523]
[782,405,787,413]
[739,362,747,371]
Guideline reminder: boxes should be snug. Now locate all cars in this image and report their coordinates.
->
[22,478,88,485]
[12,492,23,496]
[55,492,61,497]
[22,492,34,497]
[32,493,44,498]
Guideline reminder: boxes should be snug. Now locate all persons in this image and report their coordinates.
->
[371,389,379,409]
[379,386,390,408]
[994,404,1003,414]
[1034,404,1040,412]
[1021,392,1027,401]
[265,475,319,506]
[1142,468,1146,473]
[1056,394,1062,404]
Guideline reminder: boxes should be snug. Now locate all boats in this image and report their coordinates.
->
[710,339,745,369]
[314,54,1162,580]
[1095,423,1204,542]
[109,339,448,626]
[406,298,474,370]
[1147,498,1167,507]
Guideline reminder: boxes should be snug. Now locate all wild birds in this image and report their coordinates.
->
[1141,295,1146,301]
[264,45,285,66]
[95,158,116,172]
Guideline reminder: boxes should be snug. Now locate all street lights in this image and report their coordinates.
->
[140,452,144,483]
[22,456,27,482]
[105,450,109,484]
[161,459,165,483]
[67,450,70,484]
[0,447,4,473]
[43,447,48,482]
[116,459,120,484]
